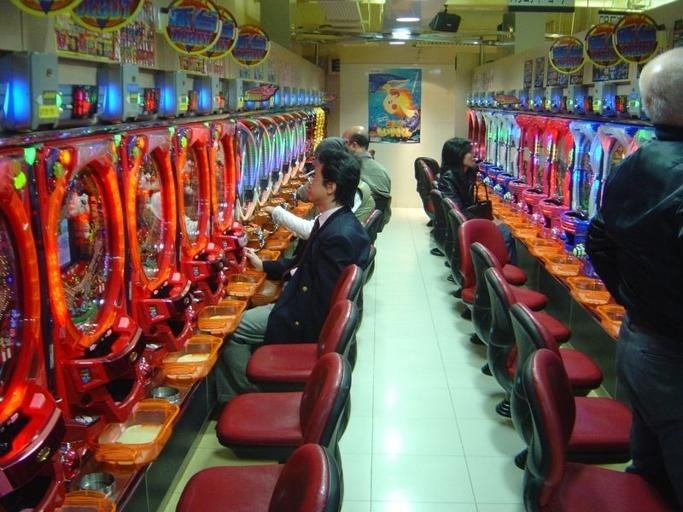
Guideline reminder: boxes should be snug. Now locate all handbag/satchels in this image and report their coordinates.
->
[467,201,493,220]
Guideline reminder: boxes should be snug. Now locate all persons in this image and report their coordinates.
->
[260,136,375,255]
[342,125,391,231]
[437,136,519,268]
[586,47,681,511]
[208,147,370,420]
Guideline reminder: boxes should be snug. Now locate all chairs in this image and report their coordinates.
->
[214,351,353,462]
[508,302,637,470]
[470,240,571,377]
[485,268,603,420]
[174,444,345,512]
[327,263,365,331]
[507,347,675,512]
[449,207,530,321]
[361,243,377,286]
[244,299,360,393]
[360,177,393,233]
[459,217,548,347]
[363,208,383,246]
[414,155,468,283]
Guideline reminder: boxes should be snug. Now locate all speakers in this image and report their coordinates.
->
[429,12,461,32]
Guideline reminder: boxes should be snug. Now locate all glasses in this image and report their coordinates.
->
[307,175,330,184]
